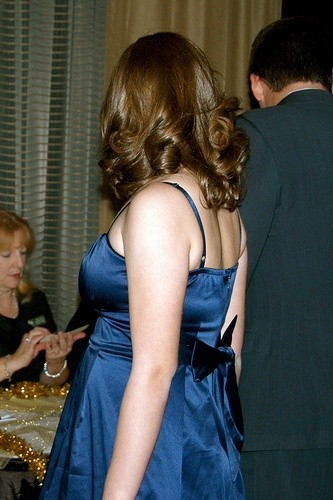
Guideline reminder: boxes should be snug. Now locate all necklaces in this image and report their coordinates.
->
[1,290,19,319]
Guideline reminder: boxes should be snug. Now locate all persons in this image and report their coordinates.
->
[235,17,333,500]
[0,209,86,388]
[39,32,252,500]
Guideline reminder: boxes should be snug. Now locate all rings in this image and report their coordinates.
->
[26,337,30,342]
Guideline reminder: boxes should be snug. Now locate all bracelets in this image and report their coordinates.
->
[3,354,11,382]
[42,360,67,378]
